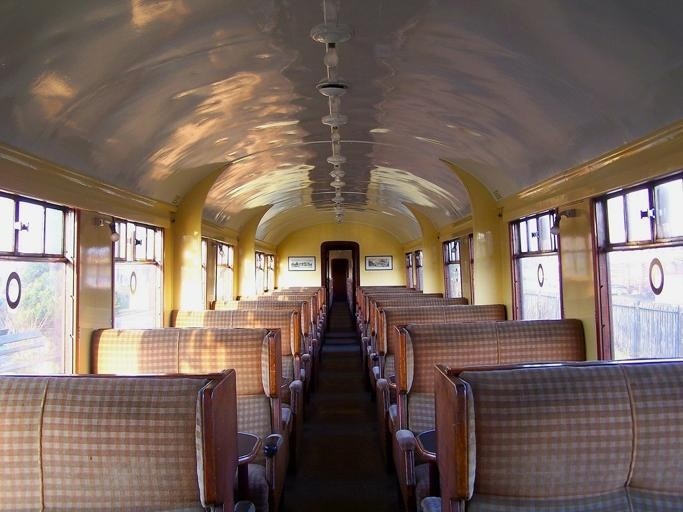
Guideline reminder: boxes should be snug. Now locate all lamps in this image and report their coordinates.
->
[415,253,420,259]
[96,216,120,243]
[310,7,352,223]
[270,255,273,263]
[211,242,224,256]
[405,255,410,261]
[258,252,262,261]
[550,209,576,236]
[451,239,463,253]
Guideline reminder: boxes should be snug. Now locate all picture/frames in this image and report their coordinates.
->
[288,255,316,271]
[364,255,393,271]
